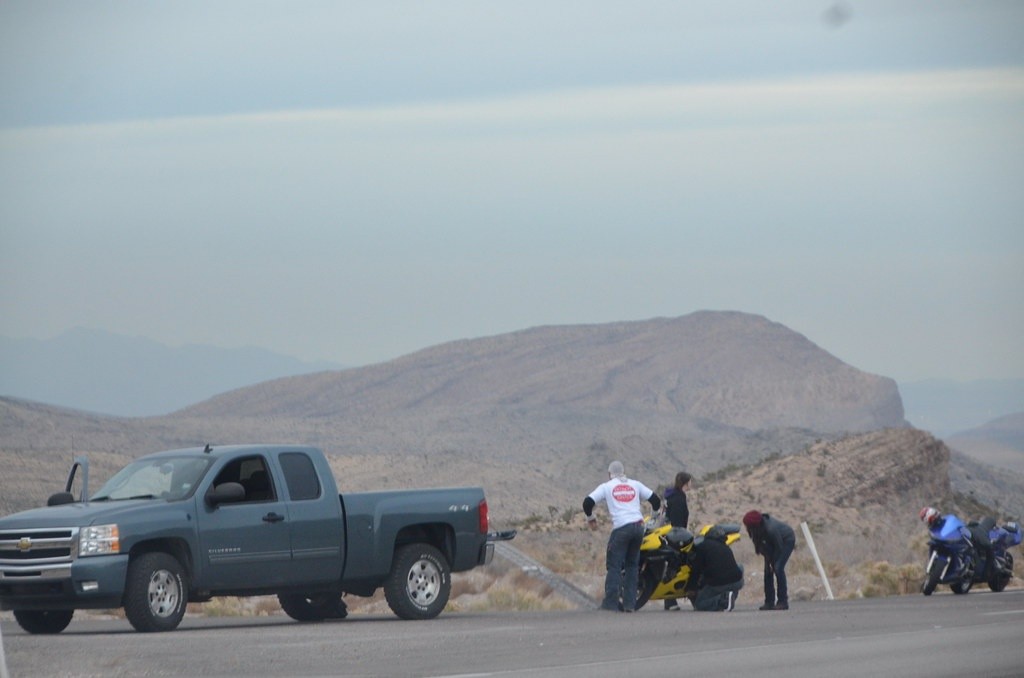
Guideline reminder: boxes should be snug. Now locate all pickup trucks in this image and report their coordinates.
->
[0,443,495,633]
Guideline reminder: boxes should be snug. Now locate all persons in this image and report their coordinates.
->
[664,472,691,609]
[689,528,744,612]
[583,461,661,612]
[743,510,795,610]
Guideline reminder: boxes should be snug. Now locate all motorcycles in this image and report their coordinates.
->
[618,510,740,611]
[919,507,1022,596]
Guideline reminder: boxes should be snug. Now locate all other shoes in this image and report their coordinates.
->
[669,604,680,611]
[624,606,635,614]
[721,591,735,612]
[760,602,788,610]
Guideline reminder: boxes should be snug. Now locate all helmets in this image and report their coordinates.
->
[1001,520,1019,533]
[918,508,942,528]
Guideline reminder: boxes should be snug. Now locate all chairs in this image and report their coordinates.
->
[247,470,272,501]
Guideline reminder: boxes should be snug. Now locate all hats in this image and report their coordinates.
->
[743,510,763,528]
